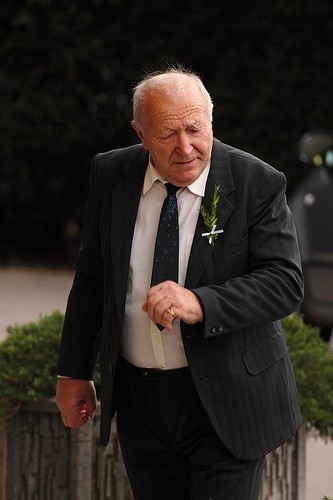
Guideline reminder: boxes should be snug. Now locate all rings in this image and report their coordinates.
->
[166,307,177,319]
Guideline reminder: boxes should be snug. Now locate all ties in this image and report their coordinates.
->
[148,184,181,333]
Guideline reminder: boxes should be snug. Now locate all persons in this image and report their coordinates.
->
[55,66,306,500]
